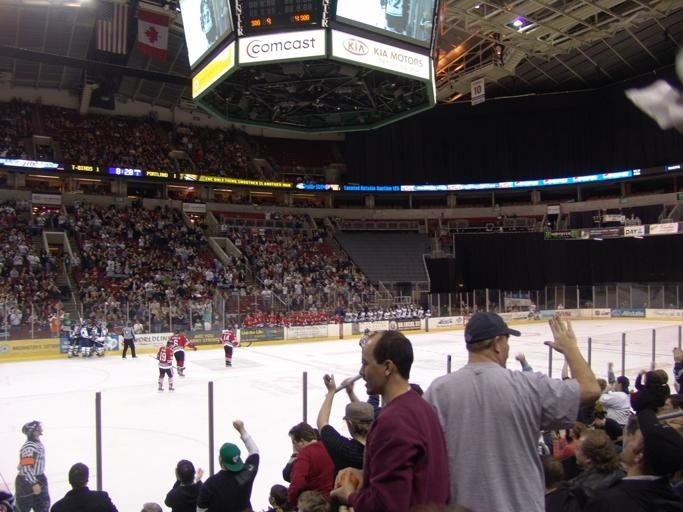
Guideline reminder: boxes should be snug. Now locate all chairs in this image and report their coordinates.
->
[341,220,420,231]
[221,217,308,235]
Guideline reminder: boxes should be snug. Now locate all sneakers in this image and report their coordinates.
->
[158,387,175,390]
[177,372,185,376]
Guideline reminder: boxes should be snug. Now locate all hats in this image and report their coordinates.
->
[343,401,374,424]
[220,443,244,472]
[465,311,520,342]
[638,409,683,475]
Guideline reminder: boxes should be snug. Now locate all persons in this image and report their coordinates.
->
[1,95,683,512]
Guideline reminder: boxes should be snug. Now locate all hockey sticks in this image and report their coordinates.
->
[148,354,184,370]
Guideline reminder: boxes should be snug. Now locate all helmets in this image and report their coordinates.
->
[22,421,39,437]
